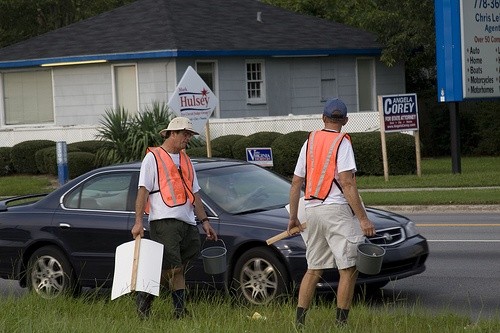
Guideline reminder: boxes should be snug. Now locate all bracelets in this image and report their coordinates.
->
[200,218,209,224]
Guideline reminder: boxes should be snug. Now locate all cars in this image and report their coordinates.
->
[0,158,430,310]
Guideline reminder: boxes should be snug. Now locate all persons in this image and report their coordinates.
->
[286,98,376,327]
[131,117,217,319]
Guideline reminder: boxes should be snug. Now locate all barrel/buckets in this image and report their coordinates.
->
[200,238,228,275]
[354,243,386,274]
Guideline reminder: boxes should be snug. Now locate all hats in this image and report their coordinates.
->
[324,99,347,118]
[159,117,199,136]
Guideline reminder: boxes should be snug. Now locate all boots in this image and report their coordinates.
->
[296,307,308,333]
[136,291,158,320]
[172,289,192,320]
[335,307,349,328]
